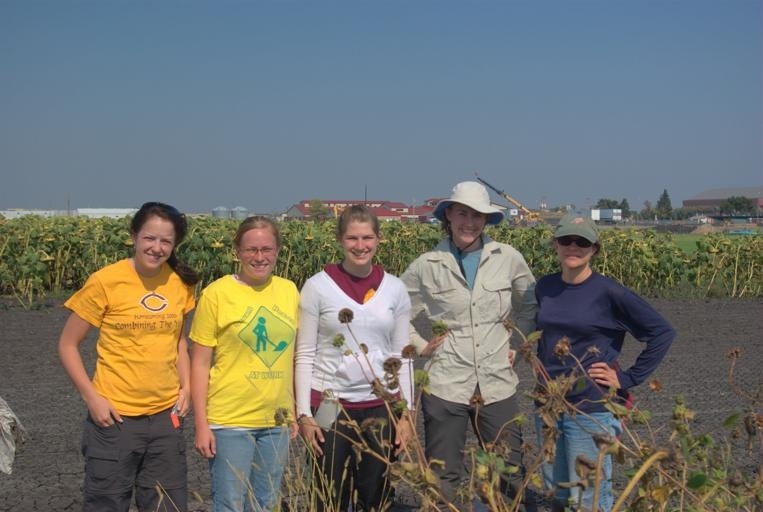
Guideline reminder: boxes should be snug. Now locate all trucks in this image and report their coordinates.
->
[591,208,622,224]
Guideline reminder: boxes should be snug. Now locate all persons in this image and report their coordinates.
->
[56,202,204,510]
[187,217,300,510]
[399,181,539,512]
[533,211,678,512]
[294,205,415,511]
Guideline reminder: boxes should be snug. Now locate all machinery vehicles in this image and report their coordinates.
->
[476,177,544,225]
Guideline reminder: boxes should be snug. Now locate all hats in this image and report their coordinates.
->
[432,180,506,226]
[553,208,601,244]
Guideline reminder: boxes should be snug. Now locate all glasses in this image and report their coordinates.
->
[558,236,592,248]
